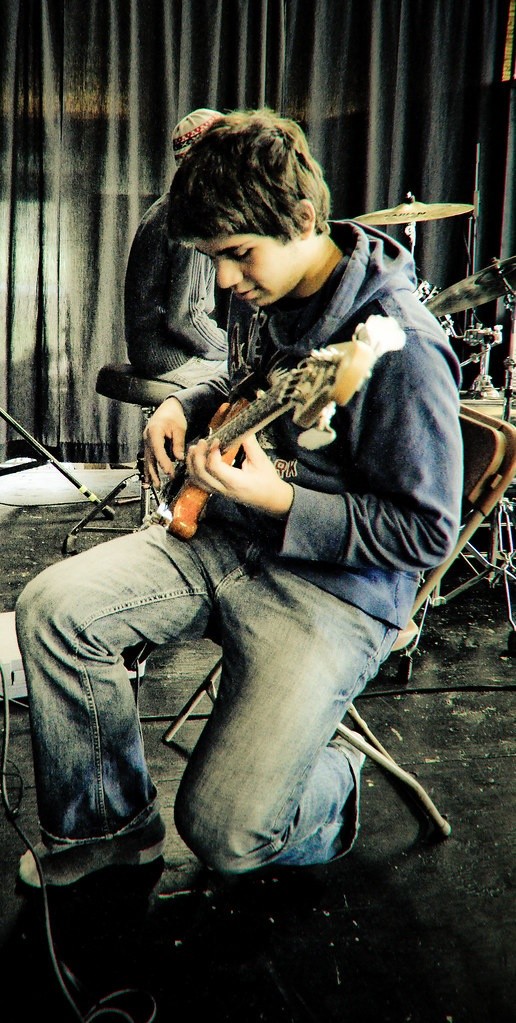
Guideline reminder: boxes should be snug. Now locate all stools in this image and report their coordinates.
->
[60,364,188,556]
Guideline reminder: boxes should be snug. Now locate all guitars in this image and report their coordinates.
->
[154,338,378,541]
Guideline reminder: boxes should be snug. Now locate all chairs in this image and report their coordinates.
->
[160,406,515,840]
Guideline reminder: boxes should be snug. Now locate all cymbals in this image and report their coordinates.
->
[353,202,478,226]
[422,254,516,318]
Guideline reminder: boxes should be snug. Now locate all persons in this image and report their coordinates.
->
[15,115,463,889]
[124,108,229,387]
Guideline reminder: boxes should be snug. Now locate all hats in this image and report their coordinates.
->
[172,108,226,166]
